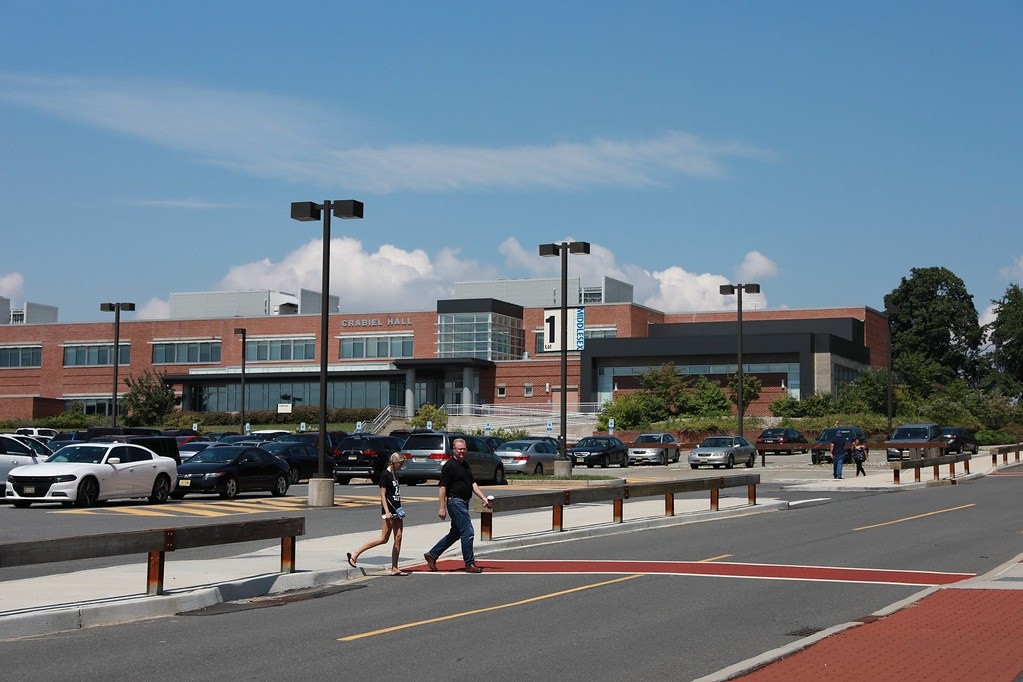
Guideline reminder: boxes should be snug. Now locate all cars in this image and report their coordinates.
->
[887,423,944,462]
[520,437,561,468]
[688,436,757,469]
[332,435,427,485]
[0,427,347,486]
[481,437,571,475]
[627,433,681,465]
[169,447,292,499]
[754,428,809,455]
[6,440,178,508]
[810,427,869,465]
[567,436,629,468]
[941,427,979,454]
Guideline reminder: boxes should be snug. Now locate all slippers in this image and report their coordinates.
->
[347,553,356,568]
[390,571,408,576]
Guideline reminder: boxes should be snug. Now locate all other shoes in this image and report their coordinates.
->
[424,553,438,571]
[465,564,481,573]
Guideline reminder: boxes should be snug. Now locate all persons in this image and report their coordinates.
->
[851,438,868,476]
[830,430,846,479]
[346,452,409,576]
[424,438,489,572]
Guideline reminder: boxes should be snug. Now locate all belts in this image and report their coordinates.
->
[451,496,461,498]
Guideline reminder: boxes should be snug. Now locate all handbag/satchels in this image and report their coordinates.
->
[396,507,405,518]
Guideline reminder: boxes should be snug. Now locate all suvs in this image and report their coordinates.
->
[397,431,504,485]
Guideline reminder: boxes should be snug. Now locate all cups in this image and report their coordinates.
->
[487,495,495,509]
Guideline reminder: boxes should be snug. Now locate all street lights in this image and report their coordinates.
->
[887,304,899,439]
[720,284,761,436]
[290,199,364,507]
[539,242,591,476]
[100,303,135,427]
[234,328,246,435]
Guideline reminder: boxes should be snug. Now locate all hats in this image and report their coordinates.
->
[836,430,841,436]
